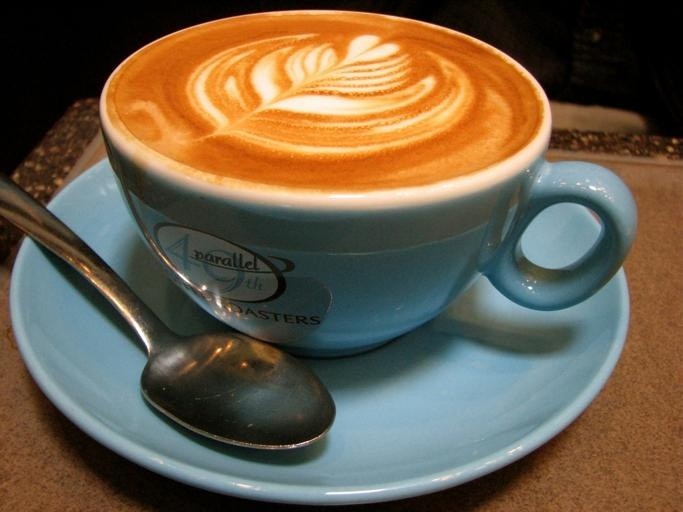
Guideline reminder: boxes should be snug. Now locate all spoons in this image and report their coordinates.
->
[0,177,338,452]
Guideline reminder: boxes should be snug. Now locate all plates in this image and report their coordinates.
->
[7,156,631,508]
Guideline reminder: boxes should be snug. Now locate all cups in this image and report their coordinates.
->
[99,10,637,365]
